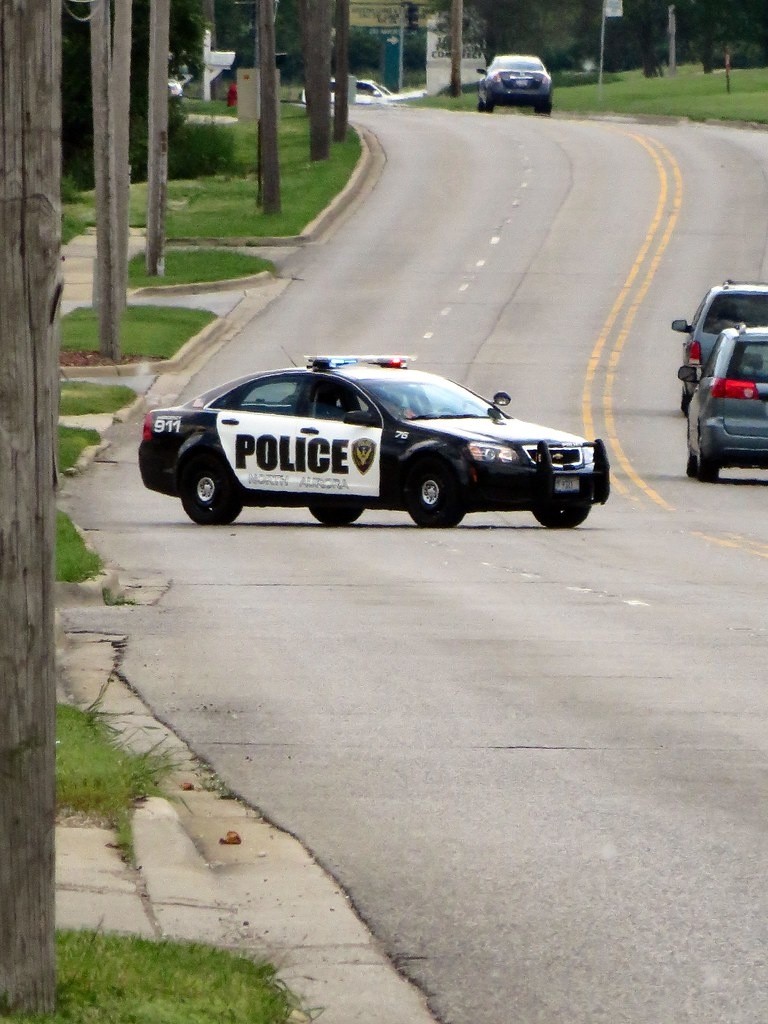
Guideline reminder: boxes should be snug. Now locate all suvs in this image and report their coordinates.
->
[671,279,768,418]
[676,322,767,483]
[299,75,393,102]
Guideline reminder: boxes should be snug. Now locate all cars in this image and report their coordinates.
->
[475,53,553,115]
[137,352,612,532]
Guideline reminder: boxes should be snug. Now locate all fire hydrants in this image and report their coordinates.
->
[226,83,237,106]
